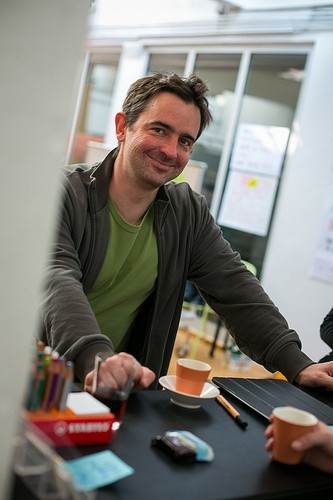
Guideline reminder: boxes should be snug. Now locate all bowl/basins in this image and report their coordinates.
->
[158,376,220,409]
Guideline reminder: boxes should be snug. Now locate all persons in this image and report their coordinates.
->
[37,73,333,401]
[265,414,333,471]
[317,305,333,363]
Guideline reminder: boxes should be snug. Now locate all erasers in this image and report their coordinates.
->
[152,429,214,461]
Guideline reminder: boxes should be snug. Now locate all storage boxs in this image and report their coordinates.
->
[21,408,115,446]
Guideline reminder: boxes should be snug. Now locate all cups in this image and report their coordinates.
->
[271,406,319,465]
[176,358,213,396]
[93,352,135,430]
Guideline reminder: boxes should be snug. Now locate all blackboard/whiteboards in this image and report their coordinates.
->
[81,141,206,197]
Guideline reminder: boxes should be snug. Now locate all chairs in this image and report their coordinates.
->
[180,257,257,363]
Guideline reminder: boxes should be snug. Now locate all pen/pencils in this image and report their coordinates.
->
[19,339,73,411]
[217,394,249,428]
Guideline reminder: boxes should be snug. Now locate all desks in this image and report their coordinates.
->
[13,376,333,500]
